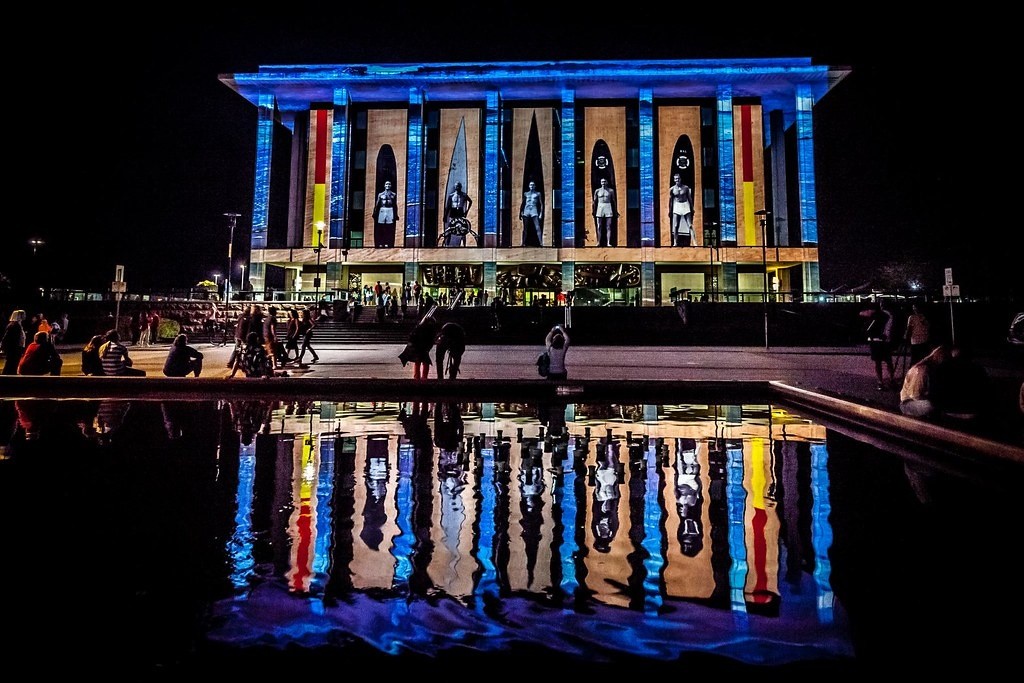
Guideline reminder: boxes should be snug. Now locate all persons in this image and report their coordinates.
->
[667,174,699,247]
[435,324,466,379]
[903,302,931,368]
[858,299,896,391]
[518,182,544,248]
[592,179,620,248]
[204,296,322,377]
[442,181,473,249]
[542,325,570,381]
[398,315,436,380]
[371,181,399,248]
[162,333,204,379]
[1005,307,1024,360]
[338,272,715,323]
[899,344,950,420]
[0,309,162,379]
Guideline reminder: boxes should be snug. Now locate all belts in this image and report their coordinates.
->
[903,399,913,405]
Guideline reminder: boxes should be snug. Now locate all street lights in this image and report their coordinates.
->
[222,211,242,344]
[314,220,328,328]
[755,209,772,351]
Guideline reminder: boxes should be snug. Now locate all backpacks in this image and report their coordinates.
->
[865,310,890,342]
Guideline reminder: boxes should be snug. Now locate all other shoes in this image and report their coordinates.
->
[889,379,896,386]
[295,359,302,363]
[877,382,884,390]
[226,363,232,369]
[272,366,277,370]
[311,357,319,362]
[281,364,285,367]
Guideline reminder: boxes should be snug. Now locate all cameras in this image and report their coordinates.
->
[556,326,560,329]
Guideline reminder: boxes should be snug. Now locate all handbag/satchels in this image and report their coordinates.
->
[534,344,552,377]
